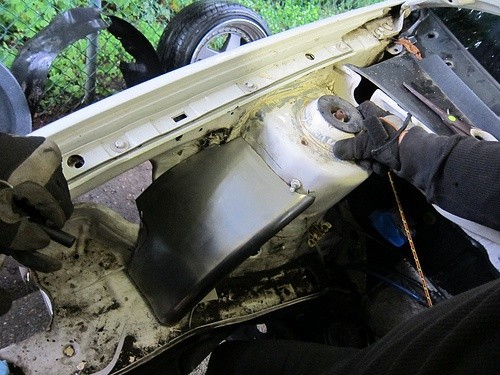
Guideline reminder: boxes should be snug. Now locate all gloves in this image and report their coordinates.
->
[333,100,402,177]
[0,131,75,251]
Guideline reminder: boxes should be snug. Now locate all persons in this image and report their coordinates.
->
[333,81,500,235]
